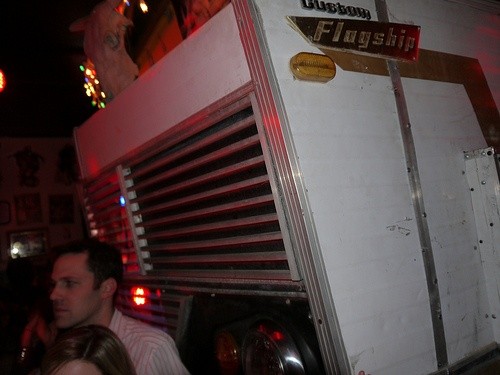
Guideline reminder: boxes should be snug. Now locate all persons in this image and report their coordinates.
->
[38,324,137,375]
[50,238,190,374]
